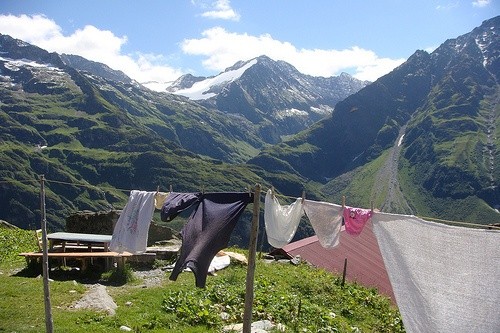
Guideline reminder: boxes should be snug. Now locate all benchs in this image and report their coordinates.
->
[19,250,133,276]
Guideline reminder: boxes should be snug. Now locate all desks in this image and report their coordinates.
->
[47,232,114,271]
